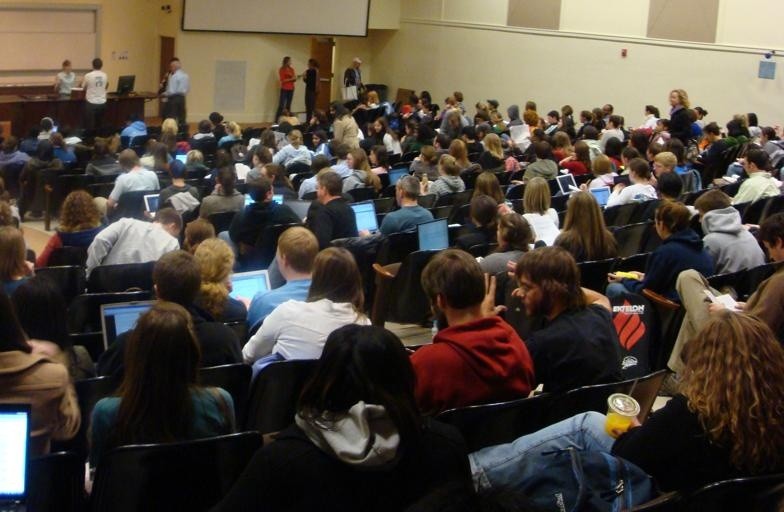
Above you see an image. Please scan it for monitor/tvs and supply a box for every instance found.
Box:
[107,75,136,96]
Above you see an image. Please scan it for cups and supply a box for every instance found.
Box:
[604,393,641,440]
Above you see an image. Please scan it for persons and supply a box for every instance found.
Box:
[521,177,560,249]
[87,208,181,282]
[37,117,53,140]
[120,115,146,136]
[592,108,606,133]
[390,90,440,123]
[543,111,561,136]
[271,129,312,168]
[768,167,784,195]
[454,194,497,248]
[328,174,435,257]
[204,150,238,191]
[368,91,380,109]
[258,164,299,199]
[643,105,660,128]
[307,168,355,218]
[159,57,179,119]
[621,149,641,175]
[207,324,478,512]
[217,178,303,272]
[329,140,341,166]
[303,58,320,123]
[562,105,573,116]
[36,191,103,271]
[628,133,646,154]
[744,198,784,264]
[330,101,359,149]
[246,130,278,163]
[628,171,683,224]
[506,104,522,127]
[694,189,765,276]
[649,119,670,145]
[472,133,505,170]
[17,139,65,217]
[93,250,244,384]
[605,137,622,167]
[522,129,545,162]
[232,144,251,181]
[665,90,690,139]
[278,109,300,126]
[54,60,76,95]
[331,144,354,180]
[475,212,534,277]
[552,191,619,263]
[577,111,596,139]
[420,153,466,199]
[342,149,382,193]
[308,130,331,161]
[236,226,318,329]
[344,57,365,97]
[368,146,392,175]
[185,219,215,251]
[688,109,701,139]
[449,139,482,189]
[663,138,690,174]
[407,249,536,418]
[400,119,418,154]
[275,56,296,122]
[409,145,440,181]
[82,58,108,136]
[308,110,329,131]
[503,151,521,173]
[0,201,19,227]
[526,101,536,111]
[139,139,156,168]
[556,117,575,137]
[687,123,721,173]
[157,119,178,152]
[723,119,750,148]
[409,125,436,154]
[580,155,619,191]
[599,115,625,152]
[524,110,540,136]
[20,125,41,154]
[433,134,448,153]
[759,127,784,167]
[352,90,371,114]
[162,60,188,123]
[551,131,573,161]
[374,118,402,156]
[11,278,97,384]
[184,150,207,172]
[746,113,761,138]
[506,141,558,195]
[0,137,32,170]
[0,225,34,280]
[606,199,716,349]
[190,237,248,323]
[246,146,273,184]
[660,210,784,396]
[314,172,359,250]
[482,246,622,394]
[606,157,658,207]
[582,126,601,156]
[696,107,707,129]
[85,141,123,175]
[76,129,96,171]
[209,112,228,143]
[728,143,759,177]
[242,247,372,359]
[620,117,630,142]
[87,302,235,498]
[93,148,160,228]
[152,144,169,169]
[729,148,779,205]
[468,309,784,495]
[200,166,245,219]
[298,154,331,199]
[242,127,255,145]
[0,285,81,458]
[603,104,613,125]
[654,152,677,178]
[0,178,10,203]
[559,140,592,176]
[469,172,505,207]
[176,119,216,152]
[49,132,74,169]
[218,121,242,148]
[646,144,662,161]
[144,160,202,221]
[440,91,508,133]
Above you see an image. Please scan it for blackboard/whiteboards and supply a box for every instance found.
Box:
[0,5,102,87]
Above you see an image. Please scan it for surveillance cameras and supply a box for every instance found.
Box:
[762,50,775,61]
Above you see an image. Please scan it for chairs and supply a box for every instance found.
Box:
[548,179,560,197]
[88,261,155,292]
[512,199,524,214]
[395,88,415,104]
[578,258,614,297]
[506,184,526,198]
[627,490,684,512]
[388,153,402,163]
[43,173,96,231]
[614,253,649,276]
[688,473,784,511]
[244,358,316,428]
[376,225,417,265]
[574,173,593,186]
[89,430,265,512]
[117,191,156,219]
[602,200,635,226]
[401,151,420,161]
[370,197,398,212]
[434,189,474,206]
[206,211,234,233]
[614,220,651,253]
[435,393,552,451]
[392,162,411,168]
[560,370,667,425]
[33,264,86,294]
[364,84,386,101]
[69,289,152,332]
[450,203,470,226]
[746,261,784,295]
[681,168,703,191]
[28,450,86,512]
[642,268,748,370]
[87,183,111,194]
[255,223,297,260]
[494,272,508,316]
[390,248,442,322]
[639,198,664,222]
[690,201,752,232]
[197,364,252,426]
[470,243,498,256]
[744,195,784,224]
[330,236,375,310]
[56,375,119,463]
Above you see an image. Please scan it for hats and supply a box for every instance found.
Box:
[487,99,498,106]
[508,105,519,120]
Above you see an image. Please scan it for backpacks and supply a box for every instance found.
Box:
[609,290,653,381]
[472,446,657,512]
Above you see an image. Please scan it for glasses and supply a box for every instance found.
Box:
[401,173,410,189]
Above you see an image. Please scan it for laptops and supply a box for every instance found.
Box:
[416,217,449,252]
[344,200,379,237]
[229,270,271,312]
[387,167,409,185]
[588,186,610,207]
[53,126,58,132]
[176,155,187,164]
[0,403,32,499]
[144,194,161,217]
[100,300,160,352]
[556,173,579,195]
[244,194,284,205]
